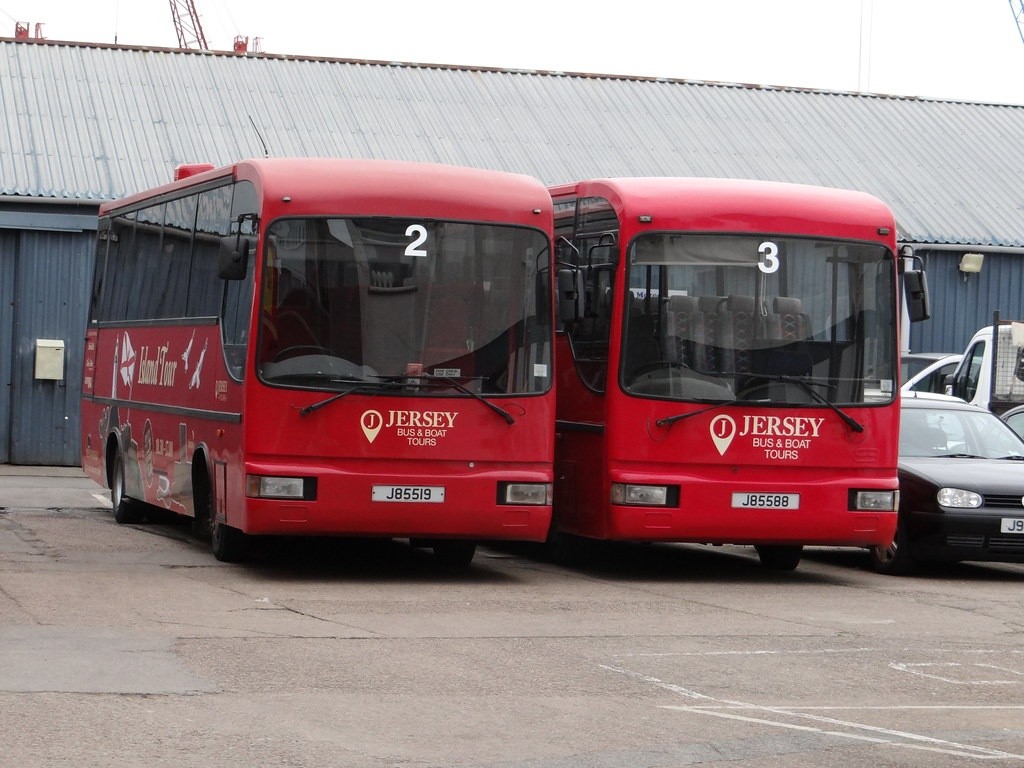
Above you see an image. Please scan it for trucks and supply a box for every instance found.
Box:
[943,311,1024,414]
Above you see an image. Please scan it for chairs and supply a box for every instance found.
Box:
[568,292,814,392]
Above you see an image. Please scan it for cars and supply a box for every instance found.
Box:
[870,390,1024,570]
[863,352,1023,402]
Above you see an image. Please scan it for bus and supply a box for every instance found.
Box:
[547,176,932,574]
[80,157,583,568]
